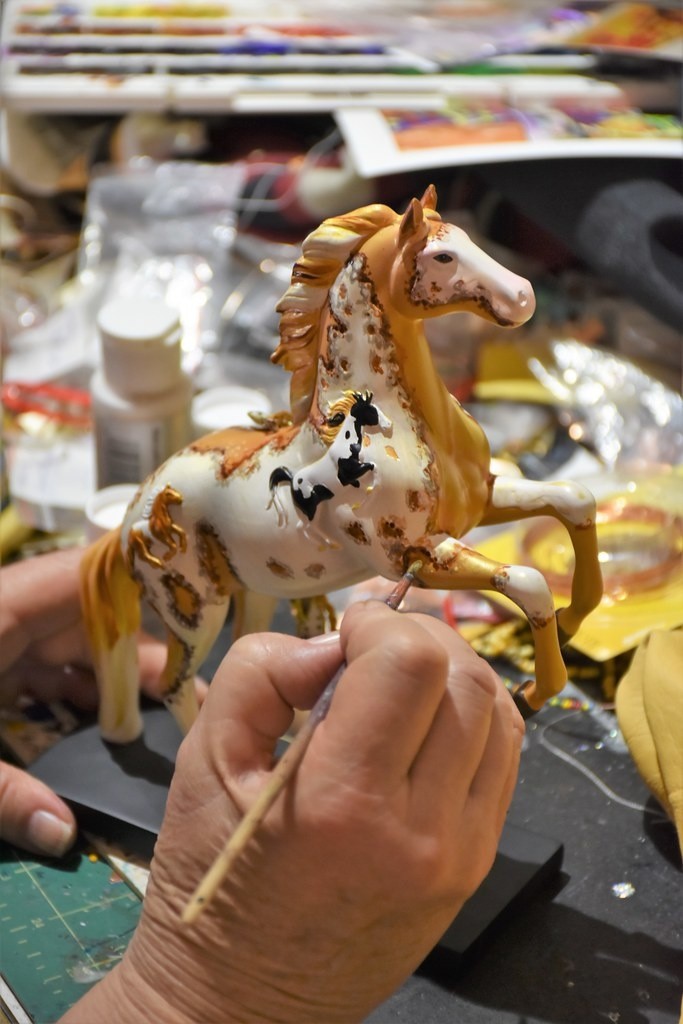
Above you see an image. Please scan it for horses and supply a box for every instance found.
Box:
[264,388,395,553]
[77,182,602,773]
[124,484,188,579]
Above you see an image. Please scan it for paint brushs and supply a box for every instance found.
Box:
[175,558,425,931]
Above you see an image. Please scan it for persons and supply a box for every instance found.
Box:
[0,531,524,1024]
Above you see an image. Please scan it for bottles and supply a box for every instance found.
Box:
[90,298,192,483]
